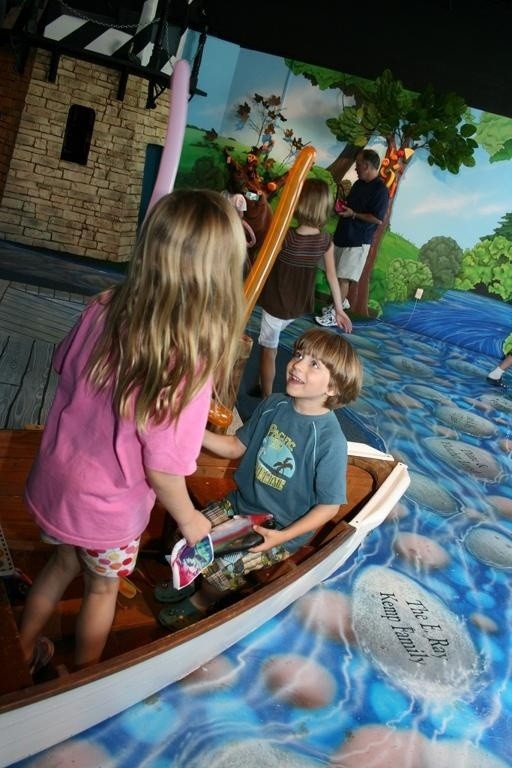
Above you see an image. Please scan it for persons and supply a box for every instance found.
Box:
[314,149,390,327]
[16,188,257,677]
[257,177,353,401]
[219,172,249,221]
[153,327,364,633]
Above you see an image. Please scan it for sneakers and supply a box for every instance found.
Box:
[486,367,504,385]
[314,298,350,327]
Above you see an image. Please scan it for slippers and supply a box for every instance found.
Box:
[155,580,205,631]
[24,637,54,676]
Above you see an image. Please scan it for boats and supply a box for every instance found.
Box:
[0,425,411,768]
